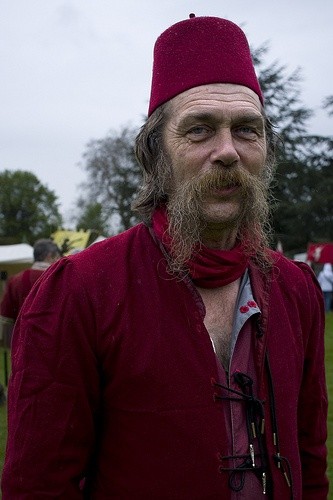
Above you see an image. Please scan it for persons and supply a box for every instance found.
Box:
[0,239,59,353]
[317,263,333,312]
[0,15,332,499]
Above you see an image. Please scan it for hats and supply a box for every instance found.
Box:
[146,12,262,105]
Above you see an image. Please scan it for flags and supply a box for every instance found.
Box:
[306,241,332,264]
